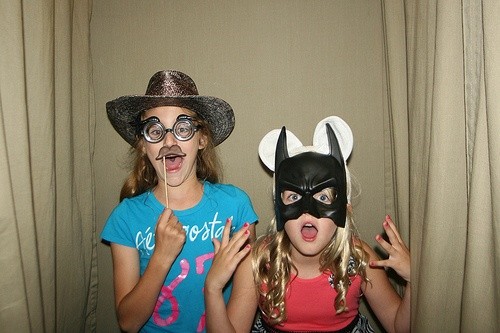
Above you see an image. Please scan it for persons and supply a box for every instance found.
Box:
[204,145,410,333]
[102,70,258,332]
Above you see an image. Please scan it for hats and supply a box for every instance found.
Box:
[106,71,235,150]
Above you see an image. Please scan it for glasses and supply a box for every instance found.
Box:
[135,116,206,143]
